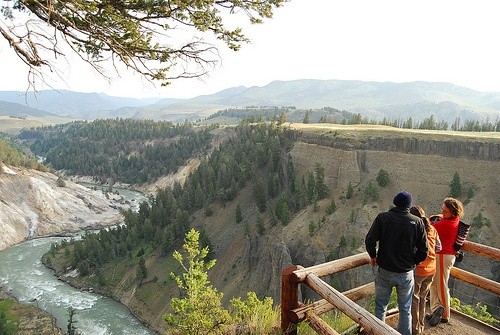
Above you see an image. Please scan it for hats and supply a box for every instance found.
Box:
[393,192,412,208]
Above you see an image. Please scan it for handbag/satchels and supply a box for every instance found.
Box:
[454,221,470,250]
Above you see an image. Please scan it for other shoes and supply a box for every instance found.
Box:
[428,307,443,326]
[426,314,447,323]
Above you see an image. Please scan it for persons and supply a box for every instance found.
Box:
[426,198,464,326]
[410,205,442,335]
[365,191,428,335]
